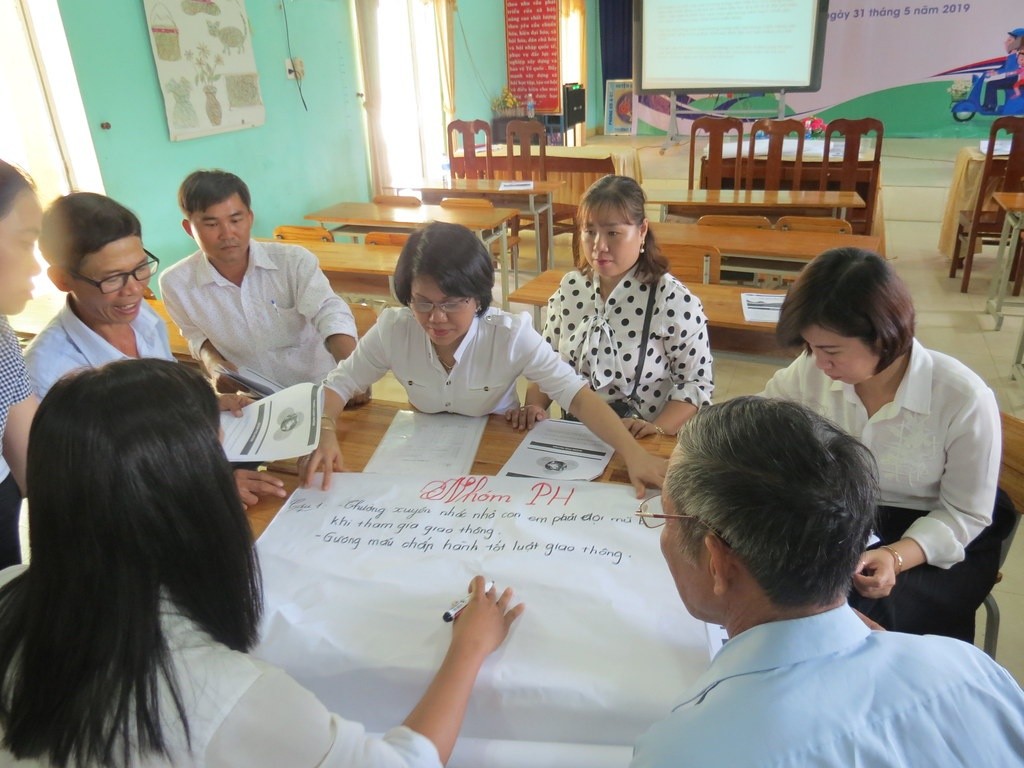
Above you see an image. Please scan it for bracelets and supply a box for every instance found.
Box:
[881,546,903,575]
[655,426,664,434]
[321,416,336,431]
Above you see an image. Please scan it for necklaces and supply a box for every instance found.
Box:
[437,354,454,369]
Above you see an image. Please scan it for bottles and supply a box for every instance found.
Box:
[804,120,812,139]
[526,94,534,119]
[751,120,764,141]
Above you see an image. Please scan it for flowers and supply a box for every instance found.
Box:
[490,85,519,116]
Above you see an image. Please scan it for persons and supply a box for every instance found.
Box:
[506,175,715,440]
[629,395,1024,768]
[297,220,669,500]
[0,160,287,572]
[158,168,371,406]
[0,358,526,768]
[753,246,1016,646]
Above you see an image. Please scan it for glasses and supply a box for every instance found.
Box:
[406,294,472,313]
[634,493,735,551]
[62,246,160,294]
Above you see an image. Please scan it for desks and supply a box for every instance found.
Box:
[238,182,889,364]
[456,143,639,217]
[984,189,1024,331]
[697,140,882,225]
[181,400,713,721]
[936,142,1023,266]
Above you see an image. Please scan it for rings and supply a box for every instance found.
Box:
[520,406,525,411]
[302,457,311,461]
[640,420,645,423]
[859,560,865,566]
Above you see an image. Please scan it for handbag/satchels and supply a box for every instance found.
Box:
[562,402,648,424]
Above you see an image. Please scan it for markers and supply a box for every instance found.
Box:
[443,580,495,622]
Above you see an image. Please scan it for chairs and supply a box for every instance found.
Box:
[270,106,885,290]
[946,116,1024,292]
[974,409,1024,658]
